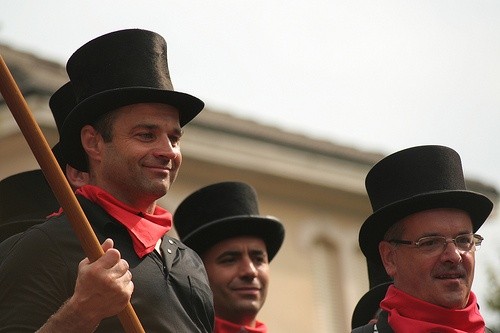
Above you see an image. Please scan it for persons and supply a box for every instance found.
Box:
[0,29,216,333]
[172,181,286,333]
[351,145,495,333]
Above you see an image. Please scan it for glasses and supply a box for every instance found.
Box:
[379,233,485,257]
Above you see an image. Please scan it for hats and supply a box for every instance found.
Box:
[48,29,205,172]
[173,180,285,263]
[357,145,496,263]
